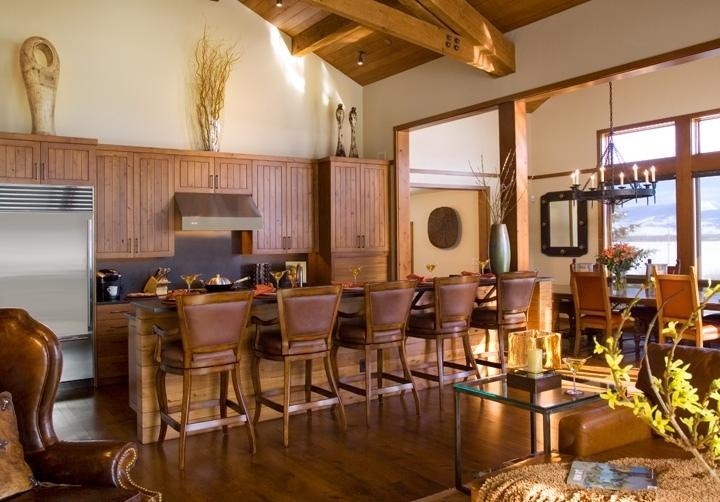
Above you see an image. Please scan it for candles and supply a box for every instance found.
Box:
[632,164,639,181]
[590,174,596,188]
[643,169,650,183]
[618,171,625,185]
[575,169,581,184]
[571,173,575,185]
[598,165,605,182]
[650,165,656,182]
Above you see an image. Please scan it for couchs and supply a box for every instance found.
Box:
[0,305,162,502]
[472,342,720,501]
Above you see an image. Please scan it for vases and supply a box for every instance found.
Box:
[594,243,648,291]
[610,271,627,296]
[490,223,511,274]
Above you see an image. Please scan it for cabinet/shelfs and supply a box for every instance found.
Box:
[94,143,176,260]
[177,151,252,196]
[312,253,398,288]
[249,155,321,254]
[95,302,134,391]
[0,132,97,186]
[316,155,396,253]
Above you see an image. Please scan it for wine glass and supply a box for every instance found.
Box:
[270,270,288,289]
[562,359,588,397]
[180,274,200,294]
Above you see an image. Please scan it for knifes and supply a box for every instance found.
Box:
[154,267,172,280]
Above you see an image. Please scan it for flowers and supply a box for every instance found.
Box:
[467,147,537,223]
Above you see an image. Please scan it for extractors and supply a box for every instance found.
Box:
[173,193,264,231]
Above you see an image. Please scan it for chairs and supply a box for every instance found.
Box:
[459,271,537,383]
[646,259,681,291]
[401,276,484,423]
[152,290,258,472]
[568,263,640,359]
[330,286,421,428]
[252,284,348,448]
[649,265,719,348]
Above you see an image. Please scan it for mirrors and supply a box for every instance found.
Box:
[540,190,589,257]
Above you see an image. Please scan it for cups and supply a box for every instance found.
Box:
[107,286,118,296]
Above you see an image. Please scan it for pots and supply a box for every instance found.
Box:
[205,274,250,292]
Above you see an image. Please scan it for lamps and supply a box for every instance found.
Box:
[274,0,283,8]
[358,52,363,66]
[570,81,658,213]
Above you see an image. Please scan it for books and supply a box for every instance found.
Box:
[566,460,658,493]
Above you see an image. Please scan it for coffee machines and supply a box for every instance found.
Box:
[97,269,122,303]
